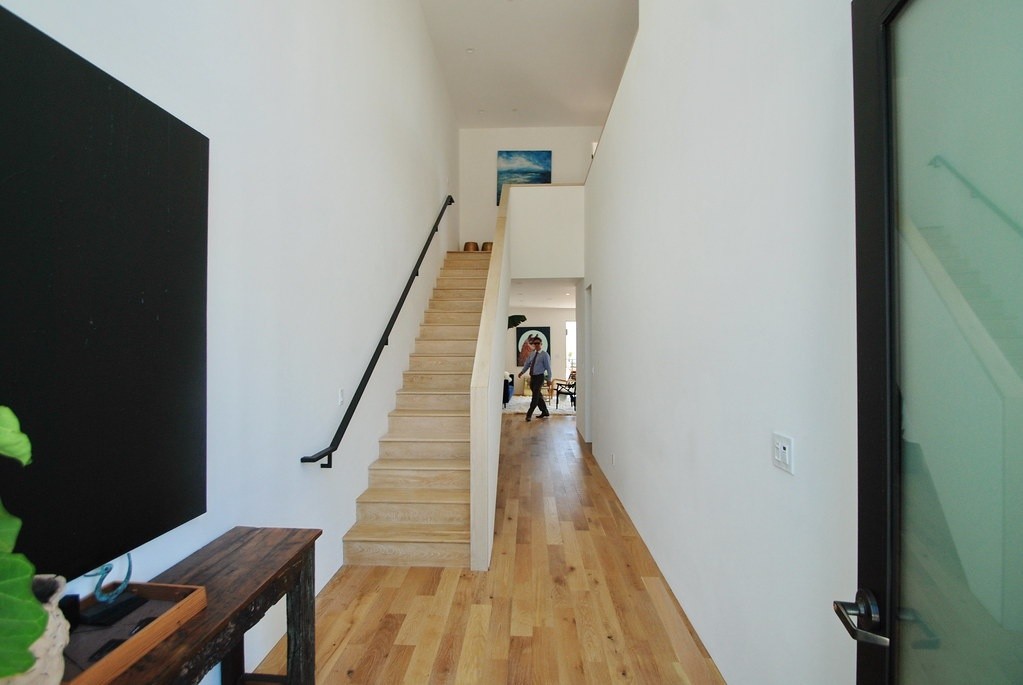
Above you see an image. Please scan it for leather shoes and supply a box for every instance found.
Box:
[536,410,549,418]
[526,415,531,421]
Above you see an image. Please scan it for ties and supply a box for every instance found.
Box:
[530,352,538,375]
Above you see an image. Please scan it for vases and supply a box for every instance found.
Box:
[464,242,479,251]
[481,241,493,251]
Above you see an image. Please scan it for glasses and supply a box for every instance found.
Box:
[534,342,541,345]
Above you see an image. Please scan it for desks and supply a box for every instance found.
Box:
[111,526,323,685]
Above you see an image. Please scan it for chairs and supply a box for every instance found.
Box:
[556,381,577,411]
[551,370,576,399]
[541,383,551,406]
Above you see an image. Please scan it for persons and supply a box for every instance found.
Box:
[517,337,553,422]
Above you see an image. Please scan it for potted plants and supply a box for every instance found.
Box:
[0,403,73,685]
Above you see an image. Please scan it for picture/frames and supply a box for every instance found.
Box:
[496,150,553,206]
[516,326,551,366]
